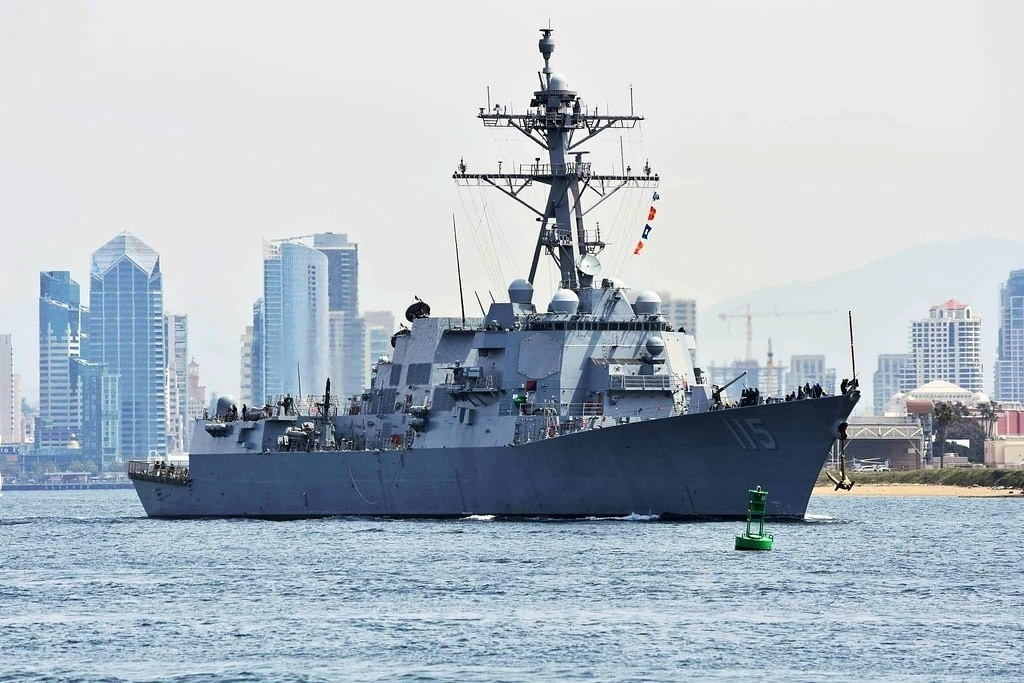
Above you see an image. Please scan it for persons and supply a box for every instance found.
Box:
[786,382,822,402]
[741,387,771,407]
[714,400,739,411]
[841,379,848,394]
[231,403,247,421]
[149,461,175,477]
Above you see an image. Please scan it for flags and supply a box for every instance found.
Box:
[634,206,657,254]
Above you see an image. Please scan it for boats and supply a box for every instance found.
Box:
[129,22,861,520]
